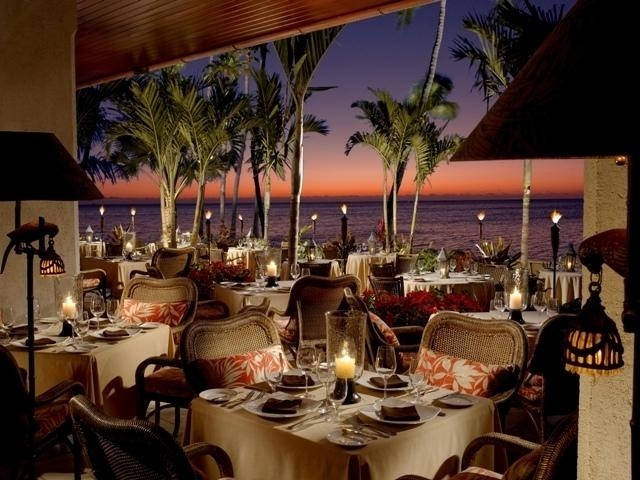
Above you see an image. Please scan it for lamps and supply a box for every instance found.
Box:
[0,131,104,417]
[564,227,626,378]
[450,0,640,480]
[565,242,576,272]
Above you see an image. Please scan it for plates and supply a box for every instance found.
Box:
[198,361,480,448]
[4,316,159,354]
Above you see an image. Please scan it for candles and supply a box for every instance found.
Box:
[62,292,78,320]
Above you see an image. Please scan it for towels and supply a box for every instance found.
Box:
[262,398,302,414]
[382,405,420,421]
[9,325,37,336]
[25,338,56,346]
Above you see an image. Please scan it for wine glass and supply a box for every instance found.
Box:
[254,263,311,288]
[489,291,561,320]
[0,295,120,346]
[356,242,384,255]
[408,258,478,279]
[263,339,429,424]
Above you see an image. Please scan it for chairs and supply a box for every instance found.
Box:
[497,314,578,445]
[0,344,84,480]
[66,394,235,480]
[392,428,570,480]
